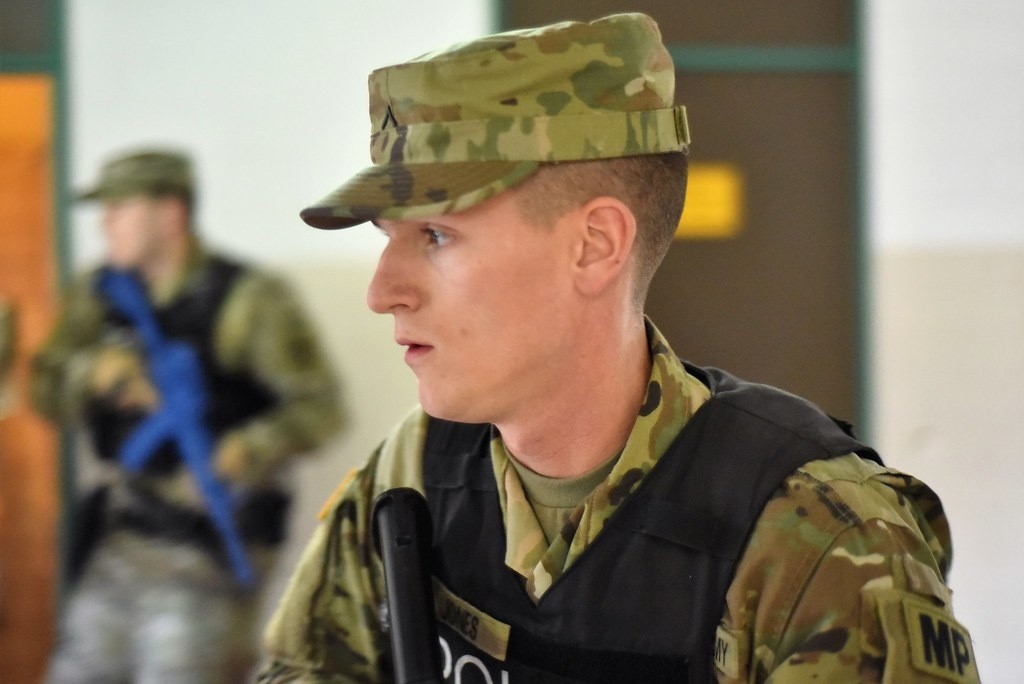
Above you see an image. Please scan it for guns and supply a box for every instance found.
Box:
[90,260,257,585]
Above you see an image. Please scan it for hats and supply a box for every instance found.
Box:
[80,151,190,199]
[300,12,692,231]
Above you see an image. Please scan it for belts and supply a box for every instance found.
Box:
[121,497,255,540]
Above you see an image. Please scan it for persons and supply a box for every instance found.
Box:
[26,151,346,684]
[244,13,981,684]
